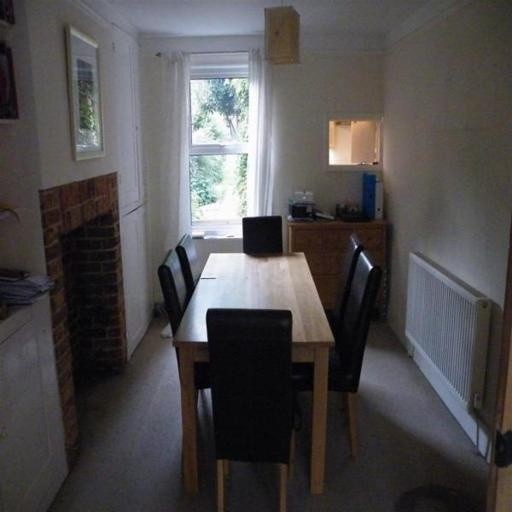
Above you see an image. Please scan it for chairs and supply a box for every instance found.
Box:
[158,249,212,478]
[295,251,382,465]
[324,233,364,328]
[206,309,295,512]
[242,216,282,253]
[175,234,204,292]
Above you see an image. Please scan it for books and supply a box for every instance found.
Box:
[0,267,56,309]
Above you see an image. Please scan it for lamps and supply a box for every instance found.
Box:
[264,7,300,65]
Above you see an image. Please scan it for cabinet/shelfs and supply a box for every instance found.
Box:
[286,216,388,323]
[0,314,71,512]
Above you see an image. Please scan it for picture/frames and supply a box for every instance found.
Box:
[63,24,105,163]
[0,0,16,28]
[0,42,20,122]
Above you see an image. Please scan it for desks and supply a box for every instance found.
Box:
[172,252,335,496]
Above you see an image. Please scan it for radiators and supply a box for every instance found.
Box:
[404,249,493,414]
